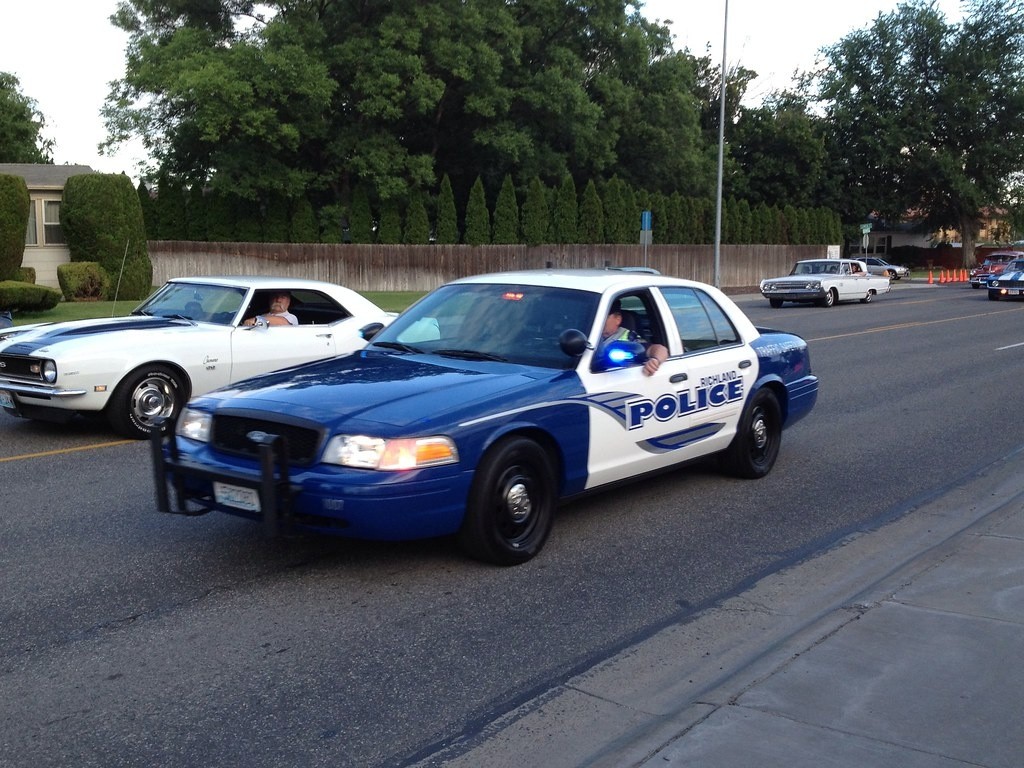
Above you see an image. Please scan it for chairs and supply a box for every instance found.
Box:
[619,310,643,338]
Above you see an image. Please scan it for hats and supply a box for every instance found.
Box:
[590,294,621,314]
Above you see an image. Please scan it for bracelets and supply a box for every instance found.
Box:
[649,356,662,365]
[253,317,257,326]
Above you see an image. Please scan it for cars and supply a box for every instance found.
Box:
[0,274,441,439]
[149,270,820,567]
[987,259,1024,300]
[760,259,891,307]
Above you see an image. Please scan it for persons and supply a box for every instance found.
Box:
[222,290,299,328]
[830,264,840,274]
[851,264,866,276]
[591,298,668,375]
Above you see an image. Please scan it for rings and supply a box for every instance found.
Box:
[247,324,250,325]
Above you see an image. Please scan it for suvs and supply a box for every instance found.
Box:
[968,251,1024,289]
[854,256,910,279]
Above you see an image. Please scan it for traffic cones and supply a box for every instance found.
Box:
[928,270,934,284]
[938,269,969,283]
